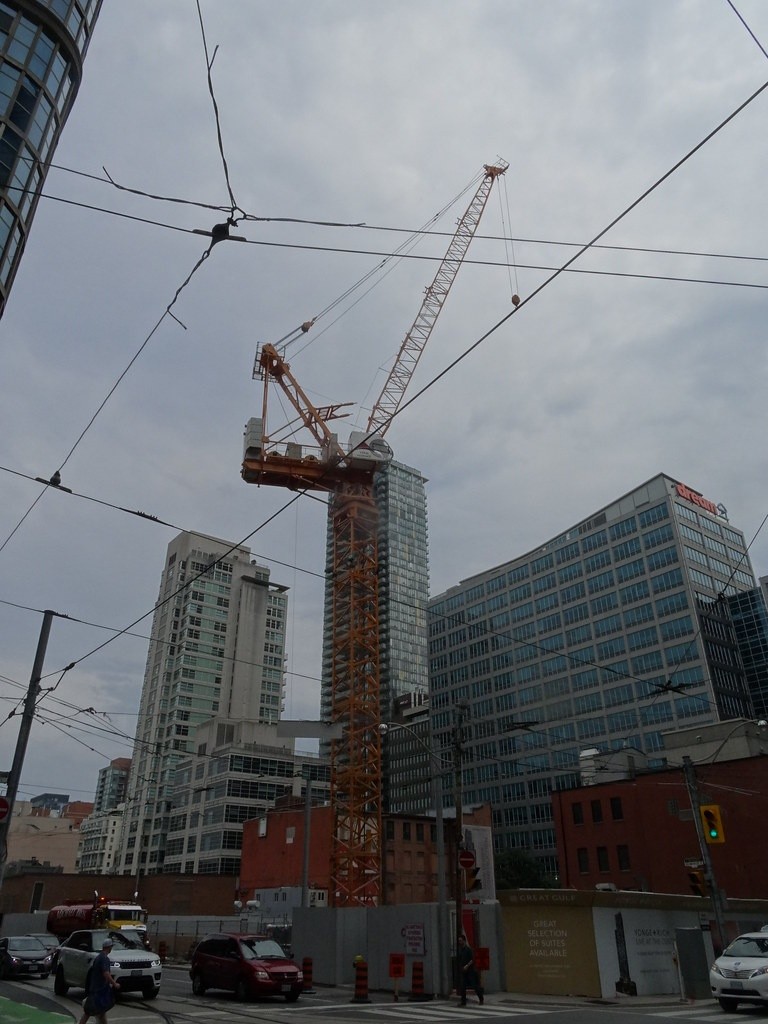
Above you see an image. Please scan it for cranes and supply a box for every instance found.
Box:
[238,156,528,461]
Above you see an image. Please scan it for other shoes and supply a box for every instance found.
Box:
[461,999,466,1005]
[479,997,485,1005]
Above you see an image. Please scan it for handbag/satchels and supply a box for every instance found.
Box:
[84,986,115,1016]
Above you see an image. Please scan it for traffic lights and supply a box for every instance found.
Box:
[699,802,725,845]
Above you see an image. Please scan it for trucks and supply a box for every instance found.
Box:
[46,890,151,951]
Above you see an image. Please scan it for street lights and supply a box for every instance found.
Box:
[678,719,767,955]
[377,719,465,939]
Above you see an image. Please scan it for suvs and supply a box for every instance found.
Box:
[53,926,162,1002]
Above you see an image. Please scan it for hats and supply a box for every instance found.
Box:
[102,938,114,948]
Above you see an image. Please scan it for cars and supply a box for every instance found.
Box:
[190,932,305,1004]
[0,936,52,979]
[23,933,61,958]
[708,925,768,1011]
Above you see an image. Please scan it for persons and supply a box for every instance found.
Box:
[456,934,484,1006]
[78,939,120,1024]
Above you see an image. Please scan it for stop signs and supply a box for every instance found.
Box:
[457,853,475,867]
